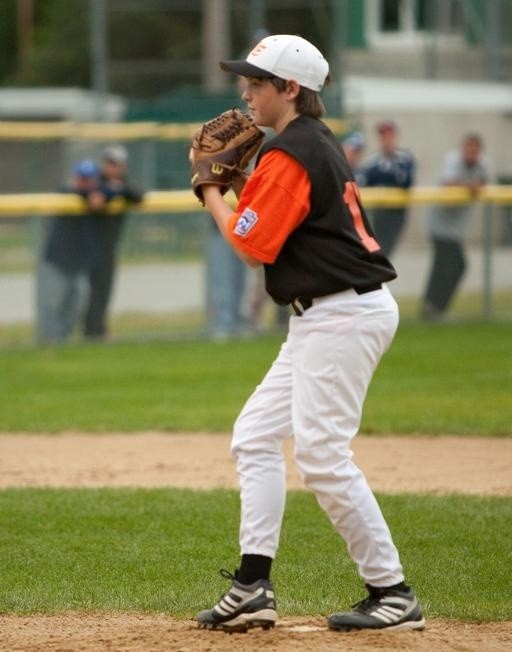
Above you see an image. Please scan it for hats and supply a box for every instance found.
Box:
[221,34,329,96]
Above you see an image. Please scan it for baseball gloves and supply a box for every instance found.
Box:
[184,104,266,206]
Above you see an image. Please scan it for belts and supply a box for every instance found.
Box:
[282,281,384,319]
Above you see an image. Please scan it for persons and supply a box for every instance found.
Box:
[341,133,373,184]
[80,144,145,338]
[205,208,259,343]
[188,34,428,636]
[413,134,488,320]
[358,120,417,262]
[32,158,102,342]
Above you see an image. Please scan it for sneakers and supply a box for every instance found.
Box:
[195,577,278,632]
[327,587,426,632]
